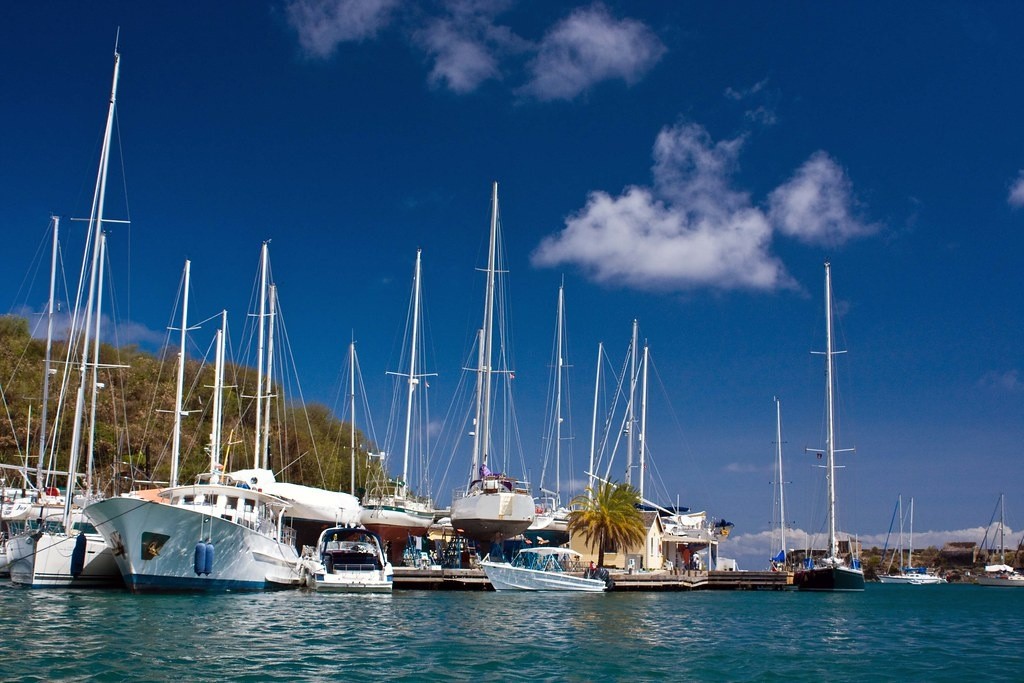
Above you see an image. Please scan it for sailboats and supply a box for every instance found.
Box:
[0,21,869,596]
[976,493,1024,586]
[876,492,950,585]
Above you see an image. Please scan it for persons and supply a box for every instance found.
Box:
[589,560,598,577]
[479,463,486,479]
[561,553,572,572]
[692,551,700,571]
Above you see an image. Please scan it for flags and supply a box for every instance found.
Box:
[768,550,786,564]
[424,381,429,388]
[509,371,515,380]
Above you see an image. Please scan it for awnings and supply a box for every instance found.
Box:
[661,534,719,572]
